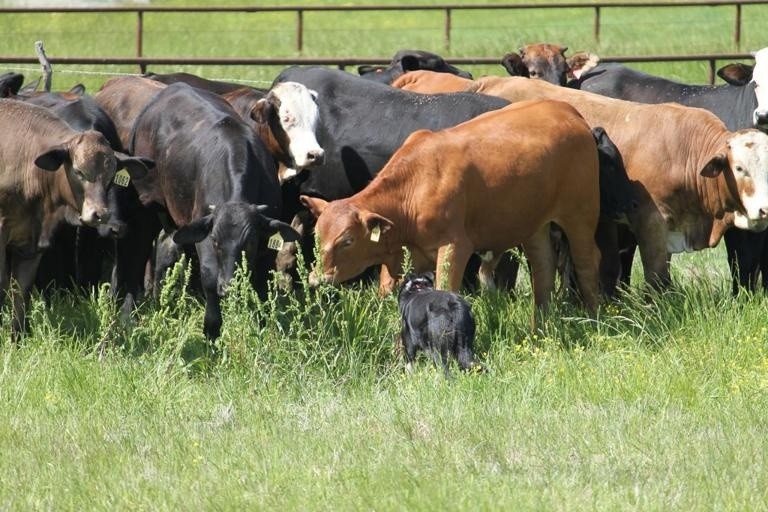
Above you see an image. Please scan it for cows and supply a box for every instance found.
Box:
[298,96,611,340]
[0,42,768,356]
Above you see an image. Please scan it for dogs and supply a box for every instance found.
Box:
[397,271,490,386]
